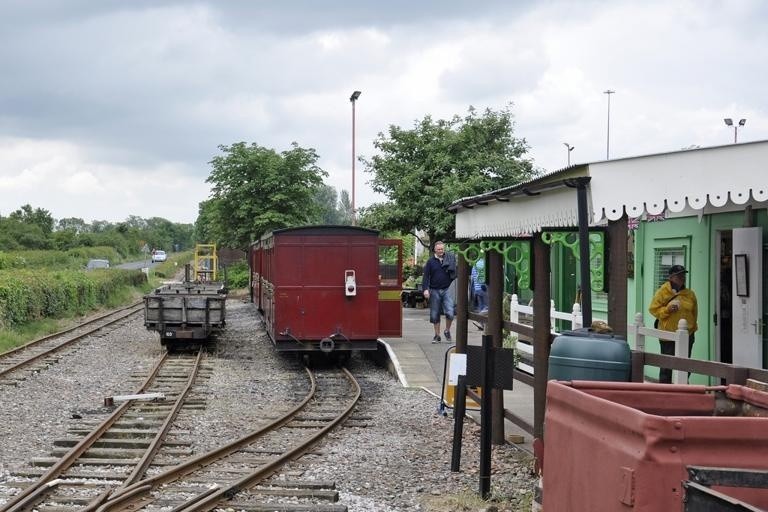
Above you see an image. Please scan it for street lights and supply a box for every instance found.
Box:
[349,91,361,226]
[723,119,747,144]
[603,90,615,160]
[563,143,574,166]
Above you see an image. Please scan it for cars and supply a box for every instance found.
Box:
[152,250,167,263]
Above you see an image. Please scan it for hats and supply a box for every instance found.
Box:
[669,265,688,276]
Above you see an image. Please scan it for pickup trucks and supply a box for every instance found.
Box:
[86,259,113,269]
[403,283,428,307]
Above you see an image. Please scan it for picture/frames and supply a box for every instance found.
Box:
[733,252,750,297]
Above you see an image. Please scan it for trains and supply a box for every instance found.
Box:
[143,243,228,348]
[245,223,403,360]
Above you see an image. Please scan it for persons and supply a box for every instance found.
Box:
[471,258,486,309]
[648,265,699,384]
[422,240,458,345]
[472,279,488,330]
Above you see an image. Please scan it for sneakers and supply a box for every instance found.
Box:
[444,330,451,341]
[432,335,441,343]
[472,321,483,330]
